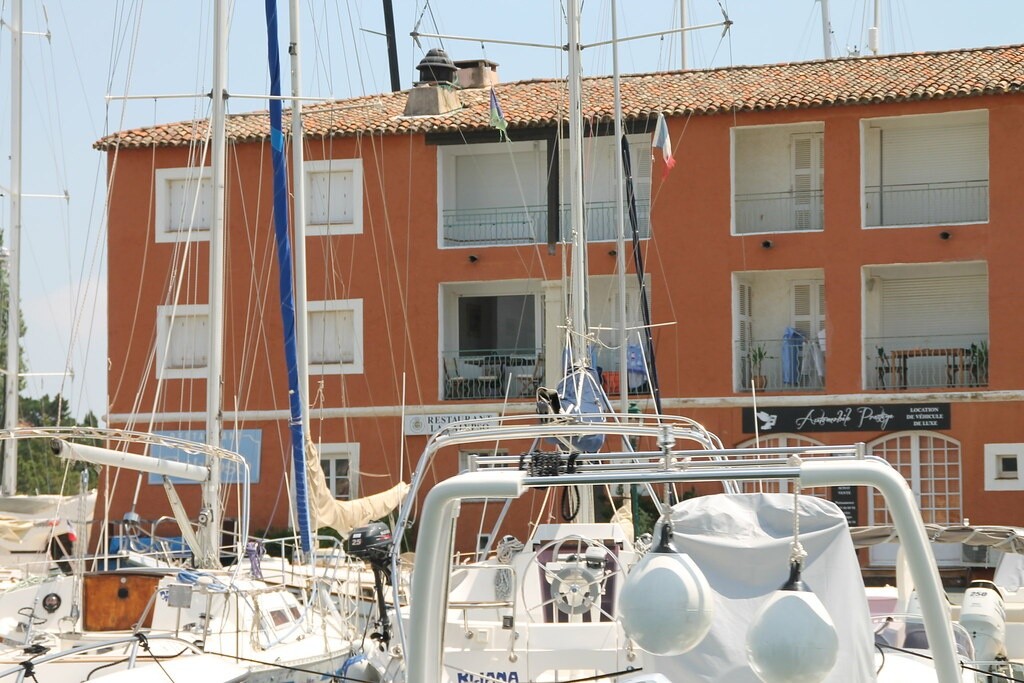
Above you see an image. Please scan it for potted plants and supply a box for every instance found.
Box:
[751,343,768,391]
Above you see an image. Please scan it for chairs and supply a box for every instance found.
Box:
[946,343,978,387]
[874,346,908,391]
[444,353,544,399]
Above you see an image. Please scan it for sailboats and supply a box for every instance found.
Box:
[1,0,1024,683]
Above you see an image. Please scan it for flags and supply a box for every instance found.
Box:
[652,112,677,168]
[488,87,508,131]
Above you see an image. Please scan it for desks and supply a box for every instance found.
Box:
[466,356,537,397]
[891,348,974,391]
